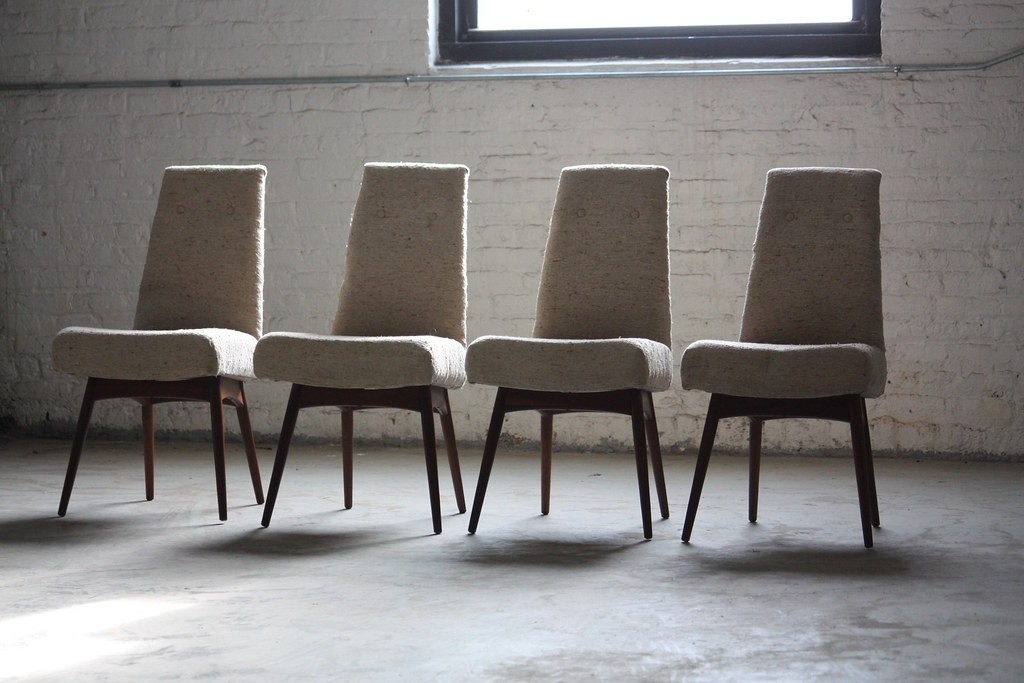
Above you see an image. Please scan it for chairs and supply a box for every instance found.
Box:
[463,164,674,541]
[253,161,473,534]
[680,168,888,549]
[49,164,268,525]
